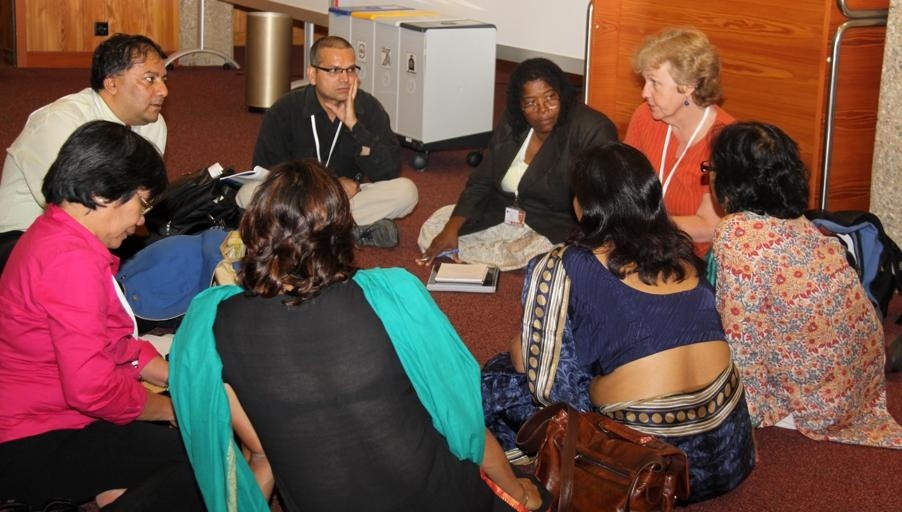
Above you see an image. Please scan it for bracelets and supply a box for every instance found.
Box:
[249,450,267,460]
[517,478,531,508]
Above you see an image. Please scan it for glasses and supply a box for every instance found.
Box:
[131,187,156,216]
[519,92,561,114]
[699,159,716,173]
[309,63,362,77]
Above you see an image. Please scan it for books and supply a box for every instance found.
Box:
[219,165,272,184]
[428,260,499,295]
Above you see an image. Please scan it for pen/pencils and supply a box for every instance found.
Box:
[420,249,460,262]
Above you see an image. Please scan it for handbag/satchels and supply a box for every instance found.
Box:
[532,406,693,512]
[144,162,244,239]
[808,208,894,327]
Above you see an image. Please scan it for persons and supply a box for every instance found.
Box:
[235,35,419,249]
[415,55,618,269]
[704,122,902,451]
[482,139,756,507]
[623,25,739,258]
[213,161,556,511]
[0,120,209,511]
[0,34,170,275]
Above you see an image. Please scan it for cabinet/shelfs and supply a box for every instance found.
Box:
[12,0,180,69]
[581,0,890,220]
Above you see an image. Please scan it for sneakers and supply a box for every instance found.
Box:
[350,217,401,251]
